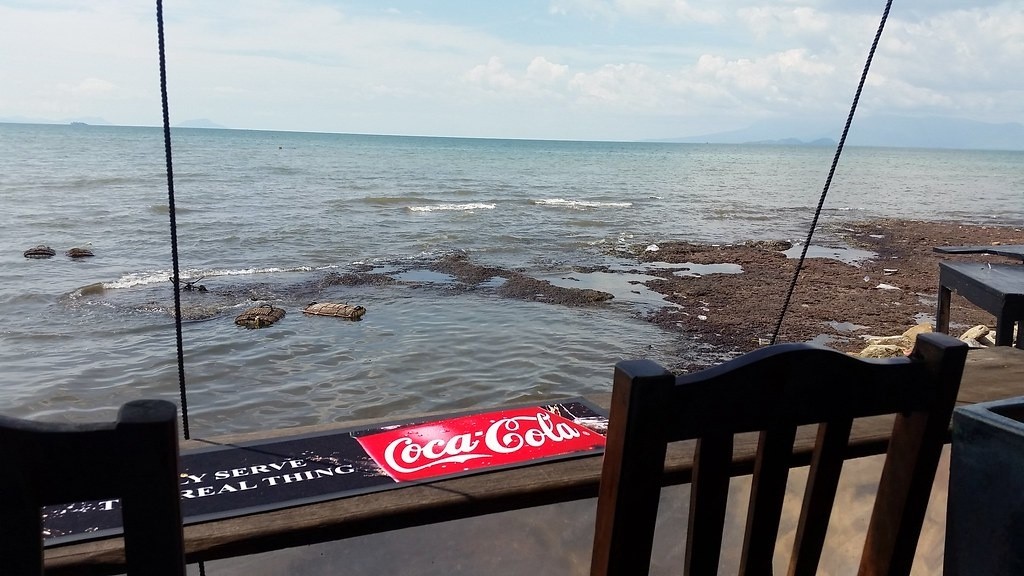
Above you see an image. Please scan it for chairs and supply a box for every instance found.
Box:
[0,400,186,576]
[590,331,970,576]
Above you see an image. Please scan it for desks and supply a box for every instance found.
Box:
[931,244,1024,349]
[42,345,1024,576]
